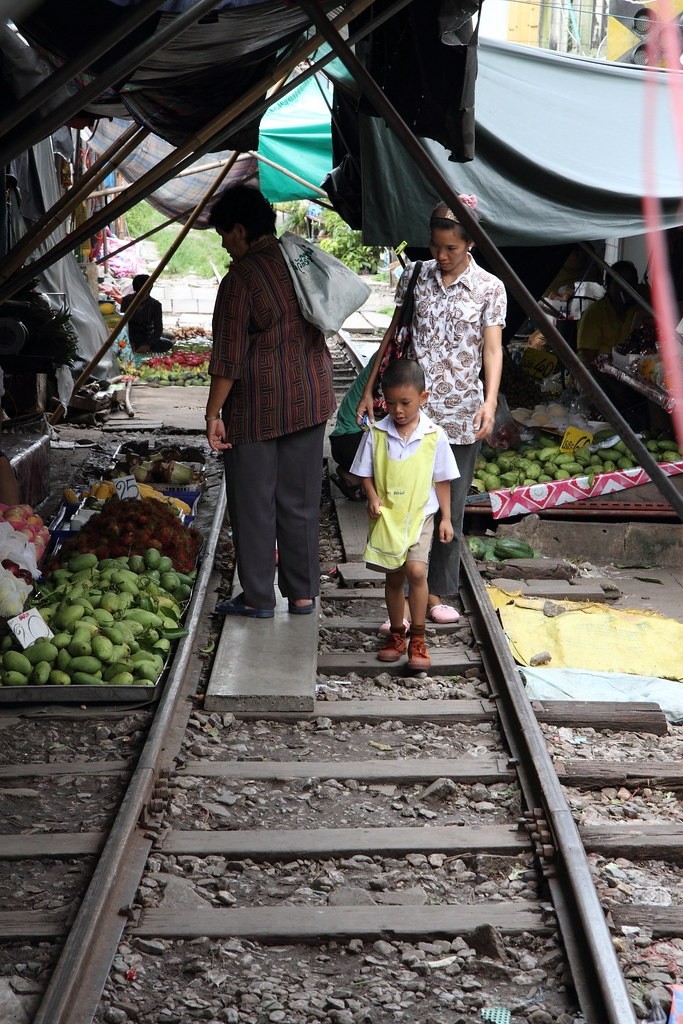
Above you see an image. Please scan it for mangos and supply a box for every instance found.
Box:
[0,549,197,687]
[456,427,682,493]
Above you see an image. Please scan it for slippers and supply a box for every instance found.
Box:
[427,604,459,624]
[287,597,316,614]
[329,472,366,501]
[216,592,276,618]
[379,618,409,635]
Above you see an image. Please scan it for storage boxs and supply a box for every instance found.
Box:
[611,345,657,376]
[33,444,207,585]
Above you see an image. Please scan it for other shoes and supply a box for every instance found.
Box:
[408,638,432,670]
[377,635,407,662]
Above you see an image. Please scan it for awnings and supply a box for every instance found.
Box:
[0,0,683,434]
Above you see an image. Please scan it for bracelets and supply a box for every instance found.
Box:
[205,414,220,422]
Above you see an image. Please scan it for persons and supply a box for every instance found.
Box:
[348,358,461,670]
[205,182,333,619]
[356,195,507,639]
[577,261,651,363]
[329,349,380,501]
[120,275,175,354]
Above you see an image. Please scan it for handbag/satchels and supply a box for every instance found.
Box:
[276,228,372,340]
[370,258,424,413]
